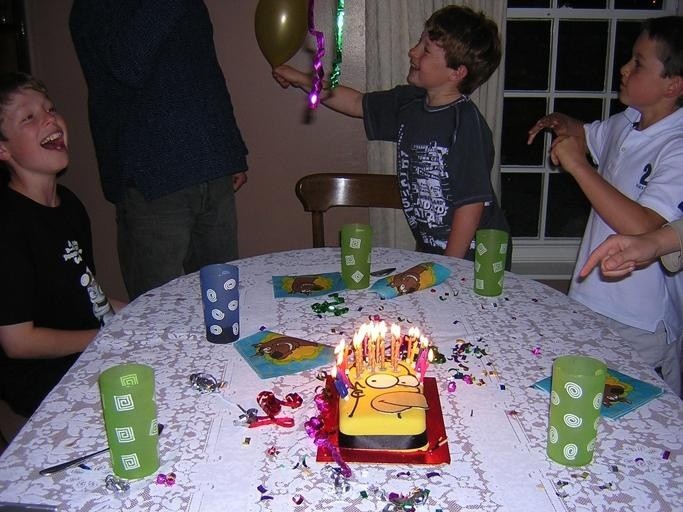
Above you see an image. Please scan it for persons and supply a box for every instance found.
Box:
[69,0,249,301]
[578,218,683,281]
[272,4,513,272]
[0,72,131,418]
[526,15,683,396]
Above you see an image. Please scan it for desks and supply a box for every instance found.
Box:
[0,247,683,512]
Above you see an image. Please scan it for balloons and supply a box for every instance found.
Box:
[253,0,309,69]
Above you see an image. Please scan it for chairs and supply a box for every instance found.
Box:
[296,173,404,248]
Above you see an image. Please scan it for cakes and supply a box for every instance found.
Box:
[338,360,431,452]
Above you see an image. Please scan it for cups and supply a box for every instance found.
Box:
[340,222,374,291]
[473,227,510,297]
[544,355,608,468]
[97,363,161,480]
[199,263,240,344]
